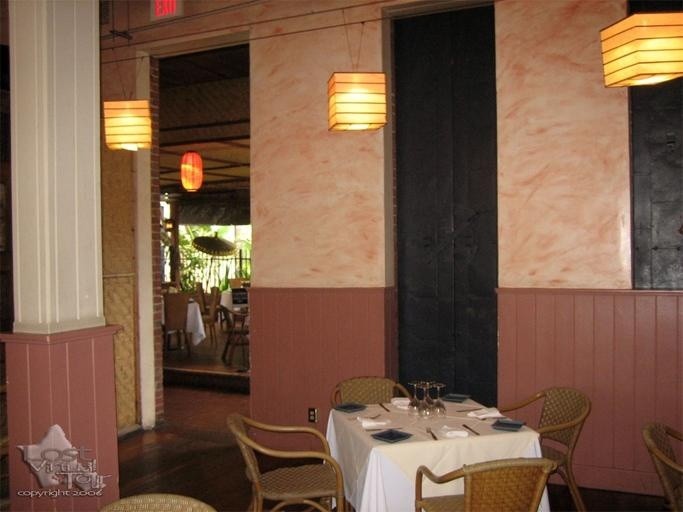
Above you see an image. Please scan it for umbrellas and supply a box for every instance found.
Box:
[192,231,238,293]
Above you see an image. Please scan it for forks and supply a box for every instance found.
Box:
[348,413,380,421]
[425,426,438,440]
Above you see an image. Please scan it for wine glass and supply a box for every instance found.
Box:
[407,380,447,418]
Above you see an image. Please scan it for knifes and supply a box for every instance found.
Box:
[456,408,482,413]
[378,402,389,413]
[462,424,481,435]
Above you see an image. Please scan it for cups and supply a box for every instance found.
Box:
[240,306,248,314]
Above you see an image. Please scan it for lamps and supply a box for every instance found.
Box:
[325,7,389,132]
[103,46,151,151]
[598,0,682,90]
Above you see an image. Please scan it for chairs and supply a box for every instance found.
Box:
[643,418,683,509]
[161,275,251,376]
[329,375,412,412]
[414,457,561,512]
[224,410,345,512]
[98,492,218,512]
[501,384,592,512]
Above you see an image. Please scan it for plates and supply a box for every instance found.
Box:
[333,402,367,413]
[440,393,471,403]
[370,428,411,443]
[491,417,525,431]
[389,397,407,406]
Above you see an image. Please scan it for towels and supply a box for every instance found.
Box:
[356,415,388,426]
[468,407,502,420]
[439,425,467,438]
[390,397,410,410]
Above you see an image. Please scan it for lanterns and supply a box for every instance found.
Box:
[181,151,204,193]
[100,97,162,152]
[324,68,388,134]
[599,10,682,87]
